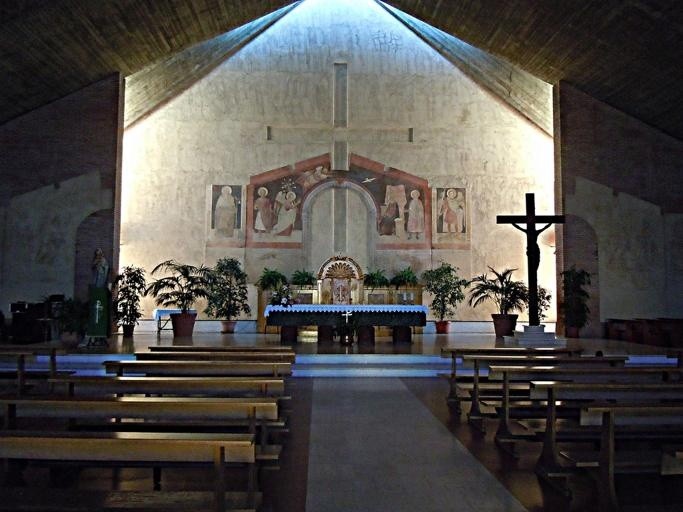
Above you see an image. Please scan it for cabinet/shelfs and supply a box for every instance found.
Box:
[363,283,424,338]
[256,284,318,335]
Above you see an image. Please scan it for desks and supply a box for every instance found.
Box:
[263,304,428,346]
[151,307,195,336]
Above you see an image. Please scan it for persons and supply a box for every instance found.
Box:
[91,247,109,286]
[440,190,463,233]
[215,186,236,236]
[272,191,301,236]
[301,165,332,191]
[407,190,424,240]
[377,193,399,235]
[254,186,273,233]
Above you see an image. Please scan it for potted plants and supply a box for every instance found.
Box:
[140,254,224,339]
[417,260,473,334]
[554,266,592,339]
[109,261,148,338]
[198,255,252,334]
[467,264,530,337]
[46,296,103,347]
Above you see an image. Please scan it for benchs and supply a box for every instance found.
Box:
[435,343,682,512]
[0,336,298,512]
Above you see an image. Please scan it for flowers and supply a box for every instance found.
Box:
[262,278,306,308]
